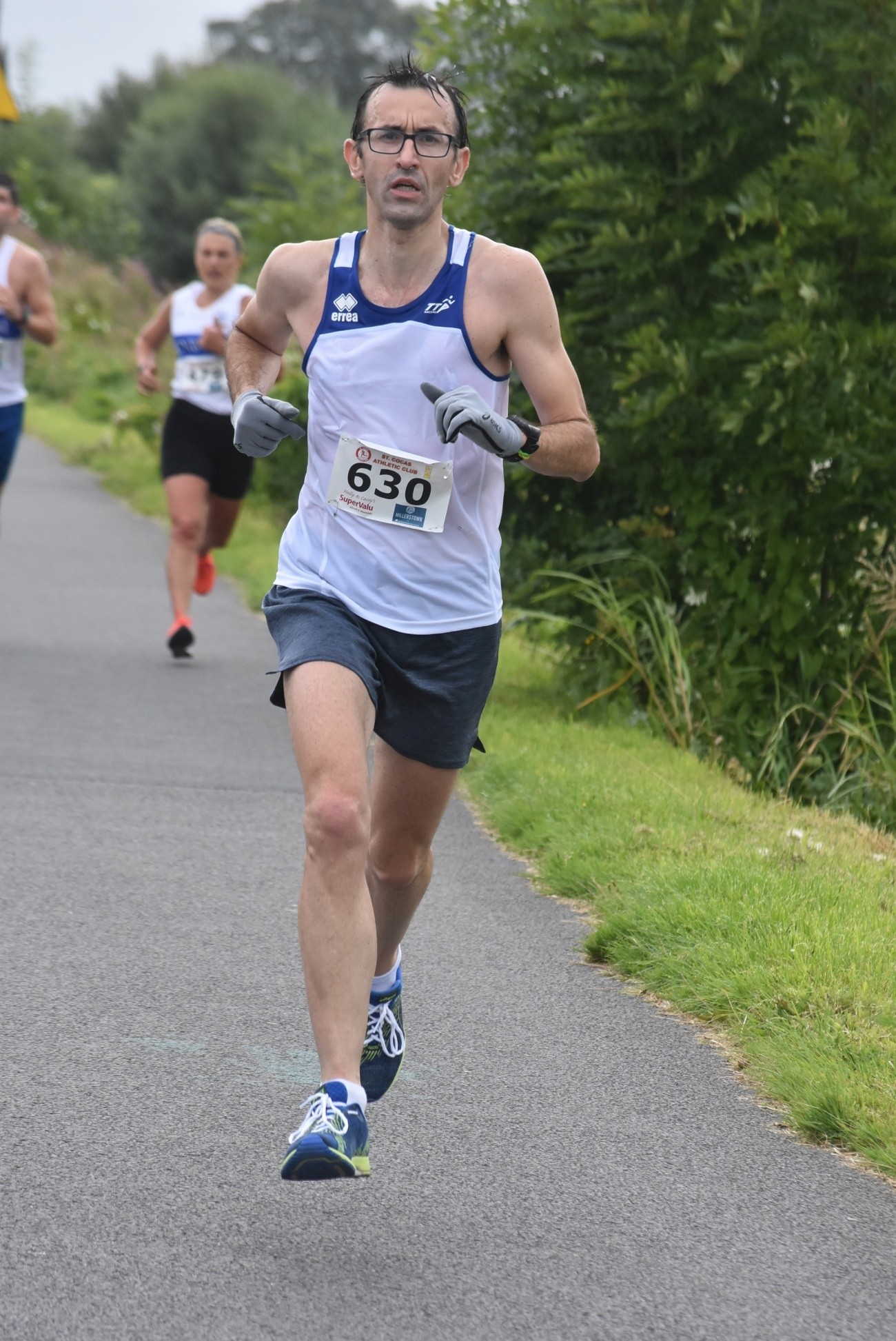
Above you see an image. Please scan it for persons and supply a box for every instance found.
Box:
[132,219,285,658]
[0,173,60,497]
[224,65,603,1179]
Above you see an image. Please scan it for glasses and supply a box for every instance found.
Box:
[197,224,242,252]
[356,127,461,158]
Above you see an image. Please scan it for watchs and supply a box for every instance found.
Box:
[496,414,542,463]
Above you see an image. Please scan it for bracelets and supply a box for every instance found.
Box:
[12,307,28,328]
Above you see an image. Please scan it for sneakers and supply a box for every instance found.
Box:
[359,963,406,1102]
[280,1081,371,1181]
[194,554,215,595]
[167,616,196,658]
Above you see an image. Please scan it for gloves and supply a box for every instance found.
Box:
[420,382,524,457]
[231,390,302,457]
